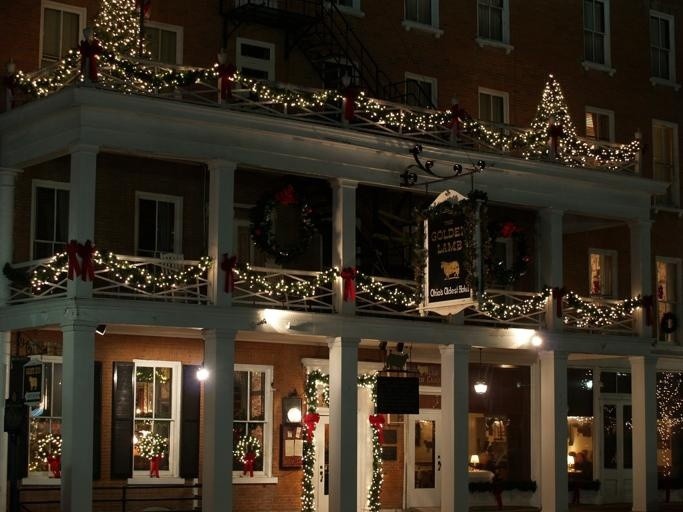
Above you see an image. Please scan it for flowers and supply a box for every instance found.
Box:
[481,220,534,288]
[247,180,318,266]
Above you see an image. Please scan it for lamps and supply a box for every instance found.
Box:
[95,326,106,336]
[469,454,480,469]
[473,348,488,395]
[195,341,209,382]
[567,455,576,472]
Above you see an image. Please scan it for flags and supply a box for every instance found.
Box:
[374,374,420,415]
[426,202,474,305]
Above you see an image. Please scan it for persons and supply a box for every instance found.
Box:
[571,453,592,471]
[472,449,502,474]
[486,446,500,465]
